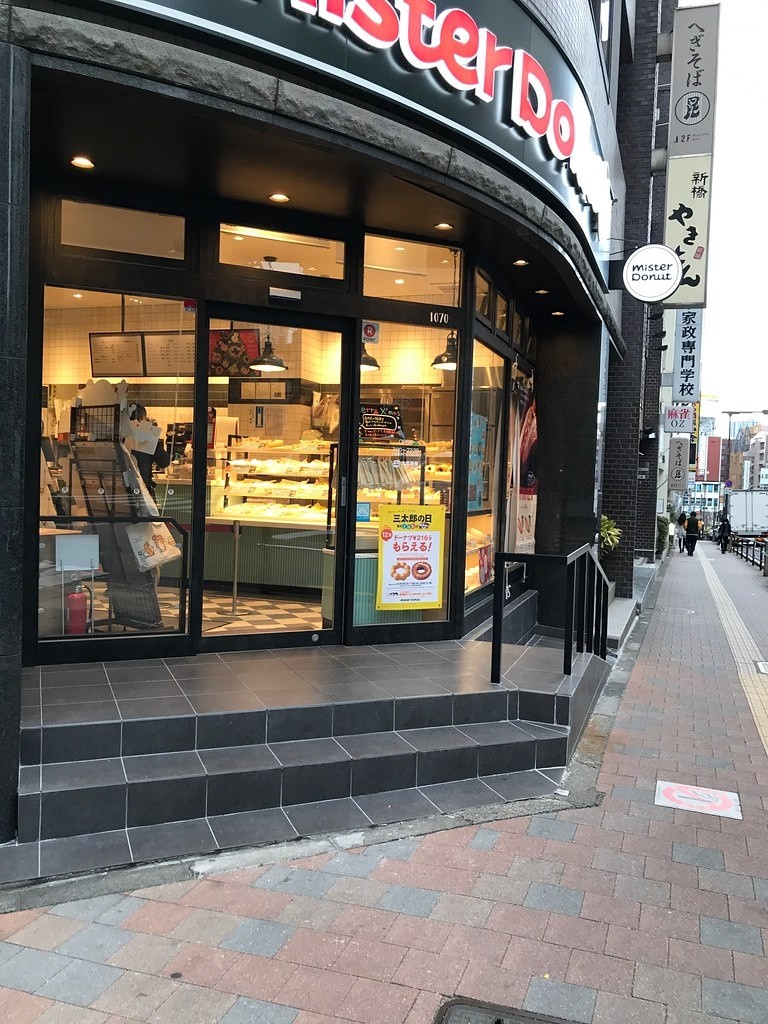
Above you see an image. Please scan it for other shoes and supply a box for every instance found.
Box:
[688,553,693,556]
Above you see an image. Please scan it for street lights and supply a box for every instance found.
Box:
[720,409,768,522]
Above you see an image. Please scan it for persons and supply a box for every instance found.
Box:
[105,403,170,596]
[675,513,686,553]
[684,511,702,556]
[718,519,731,554]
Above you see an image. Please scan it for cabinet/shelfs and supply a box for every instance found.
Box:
[224,435,453,523]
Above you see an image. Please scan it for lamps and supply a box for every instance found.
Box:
[431,331,456,370]
[361,342,380,371]
[249,325,288,372]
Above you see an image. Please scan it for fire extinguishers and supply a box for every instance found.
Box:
[64,580,92,634]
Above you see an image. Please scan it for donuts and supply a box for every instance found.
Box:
[424,464,453,473]
[411,562,432,580]
[390,562,411,581]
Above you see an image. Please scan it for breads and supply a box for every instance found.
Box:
[358,485,441,498]
[424,440,452,452]
[466,527,486,544]
